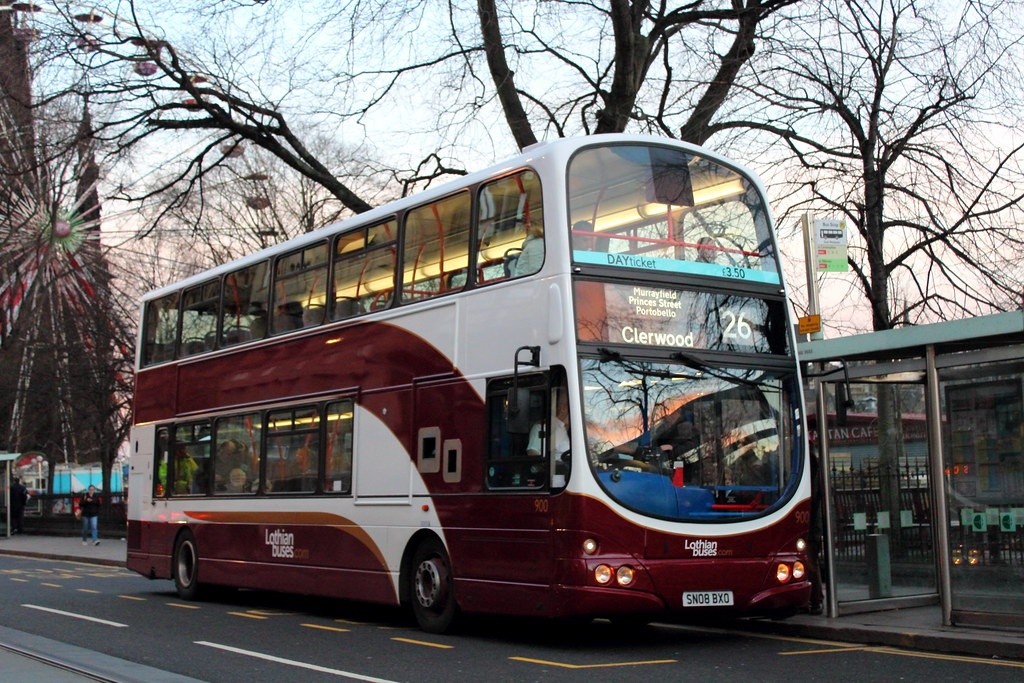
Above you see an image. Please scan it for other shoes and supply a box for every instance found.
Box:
[92,541,100,546]
[82,539,88,546]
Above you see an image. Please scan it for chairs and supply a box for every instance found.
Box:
[151,248,688,497]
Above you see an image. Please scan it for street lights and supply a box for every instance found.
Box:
[31,456,49,513]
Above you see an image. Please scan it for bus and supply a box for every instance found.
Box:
[118,129,817,636]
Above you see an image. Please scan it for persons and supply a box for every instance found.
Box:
[79,485,101,546]
[695,237,720,265]
[651,398,699,486]
[712,466,741,504]
[10,477,27,535]
[526,386,636,488]
[514,224,545,277]
[572,220,594,251]
[154,427,266,498]
